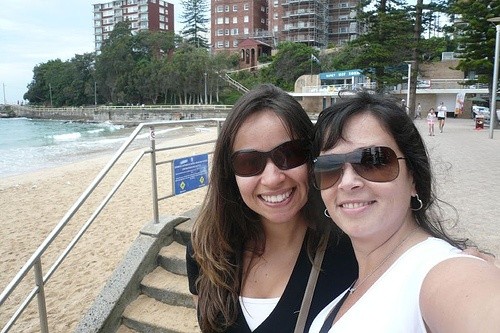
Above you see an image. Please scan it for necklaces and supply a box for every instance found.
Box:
[349,254,392,294]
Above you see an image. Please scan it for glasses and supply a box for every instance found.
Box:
[229,139,310,177]
[310,146,408,190]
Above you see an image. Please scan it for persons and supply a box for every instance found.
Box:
[415,103,423,119]
[186,84,358,333]
[437,102,447,133]
[427,108,436,136]
[310,88,500,333]
[400,98,411,111]
